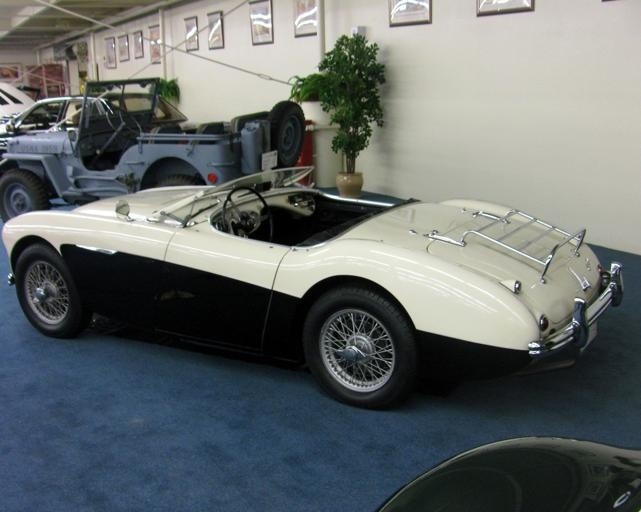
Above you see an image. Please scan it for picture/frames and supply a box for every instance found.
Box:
[117,34,130,62]
[133,30,145,60]
[0,62,24,83]
[104,36,117,69]
[475,1,535,17]
[388,0,433,29]
[206,11,225,51]
[293,0,318,39]
[248,1,275,46]
[183,16,200,51]
[148,24,161,64]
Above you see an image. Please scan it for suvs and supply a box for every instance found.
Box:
[1,78,306,222]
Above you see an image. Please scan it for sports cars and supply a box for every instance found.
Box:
[0,163,625,409]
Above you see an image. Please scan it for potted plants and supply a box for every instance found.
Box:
[289,31,387,199]
